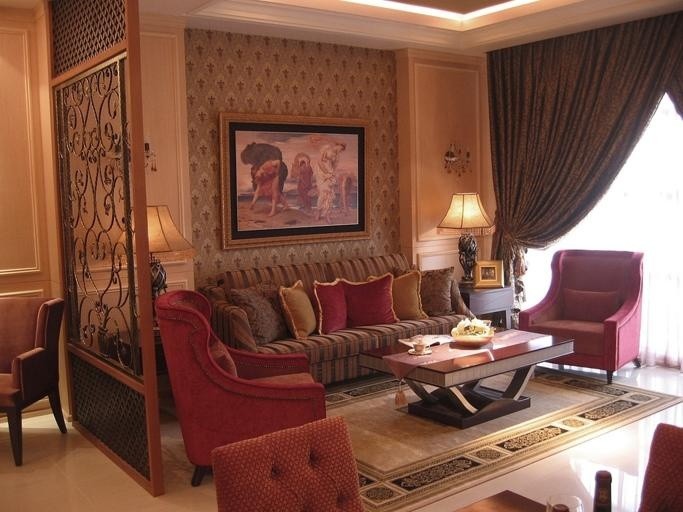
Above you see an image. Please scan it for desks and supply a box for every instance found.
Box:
[106,326,166,380]
[454,485,559,512]
[455,283,519,329]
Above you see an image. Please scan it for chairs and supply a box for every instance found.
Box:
[517,249,646,386]
[150,284,329,489]
[210,412,364,510]
[0,295,65,469]
[636,419,682,511]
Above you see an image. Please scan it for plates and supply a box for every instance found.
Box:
[409,349,430,355]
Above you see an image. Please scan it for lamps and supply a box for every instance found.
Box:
[439,140,473,177]
[117,199,192,330]
[432,191,498,286]
[142,141,158,175]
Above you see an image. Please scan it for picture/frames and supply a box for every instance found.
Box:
[216,108,376,251]
[473,259,506,289]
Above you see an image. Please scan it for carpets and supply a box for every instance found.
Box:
[295,363,681,512]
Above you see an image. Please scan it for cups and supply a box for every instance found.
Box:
[545,492,585,511]
[482,319,491,327]
[413,343,429,352]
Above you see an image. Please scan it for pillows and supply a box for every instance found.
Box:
[227,262,456,349]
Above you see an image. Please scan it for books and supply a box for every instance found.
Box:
[396,334,454,349]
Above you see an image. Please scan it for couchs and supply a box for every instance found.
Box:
[190,250,475,393]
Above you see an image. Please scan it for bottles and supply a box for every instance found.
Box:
[593,469,612,511]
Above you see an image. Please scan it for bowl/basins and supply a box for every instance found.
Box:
[451,331,493,348]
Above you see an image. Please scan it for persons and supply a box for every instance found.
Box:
[295,160,312,211]
[249,159,288,217]
[315,142,344,219]
[337,174,351,211]
